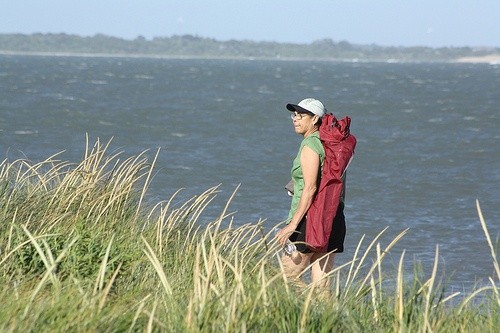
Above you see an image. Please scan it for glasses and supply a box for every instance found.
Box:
[290,113,310,120]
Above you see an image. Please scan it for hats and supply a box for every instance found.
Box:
[286,97,327,118]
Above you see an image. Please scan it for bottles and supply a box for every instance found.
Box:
[273,226,296,254]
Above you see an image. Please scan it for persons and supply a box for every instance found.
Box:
[274,98,346,287]
